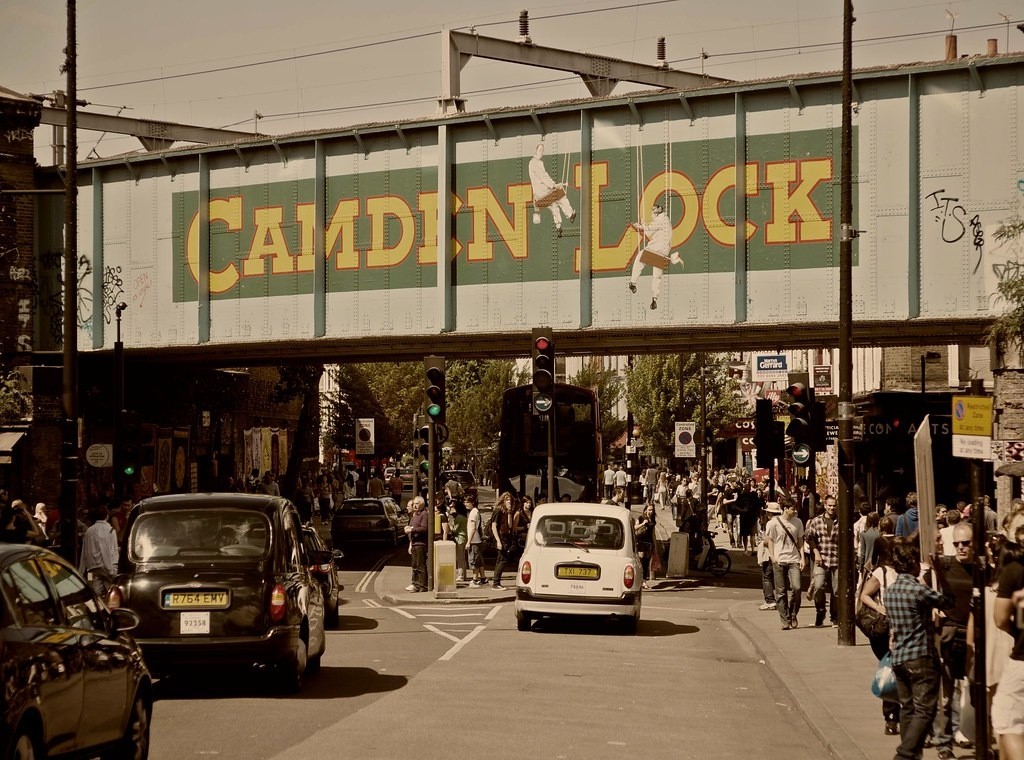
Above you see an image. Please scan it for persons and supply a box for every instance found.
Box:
[223,462,403,525]
[764,497,806,630]
[884,542,956,760]
[0,487,135,605]
[853,493,1024,760]
[601,461,815,611]
[404,474,571,592]
[806,496,839,628]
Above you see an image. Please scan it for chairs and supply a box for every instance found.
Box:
[596,521,614,540]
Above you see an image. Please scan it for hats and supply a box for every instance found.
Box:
[963,503,972,516]
[96,506,111,514]
[761,502,783,513]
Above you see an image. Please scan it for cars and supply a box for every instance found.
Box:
[0,543,155,760]
[301,520,345,631]
[105,491,336,697]
[383,465,429,493]
[514,502,653,636]
[330,494,409,551]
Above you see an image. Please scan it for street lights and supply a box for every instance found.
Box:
[699,360,748,533]
[921,351,942,421]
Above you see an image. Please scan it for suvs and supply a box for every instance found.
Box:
[435,469,479,508]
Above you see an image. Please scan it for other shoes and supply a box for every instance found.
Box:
[953,730,973,748]
[830,619,838,628]
[455,575,465,582]
[411,587,426,593]
[480,577,489,584]
[470,580,482,585]
[937,749,958,760]
[923,740,931,749]
[884,720,898,736]
[814,612,826,627]
[781,622,789,630]
[759,601,777,610]
[790,618,798,628]
[405,584,415,591]
[491,584,507,591]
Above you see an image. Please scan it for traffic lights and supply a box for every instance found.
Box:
[786,371,816,467]
[116,436,140,482]
[424,355,447,424]
[531,327,556,417]
[418,415,430,478]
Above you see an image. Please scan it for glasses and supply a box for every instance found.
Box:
[952,540,972,548]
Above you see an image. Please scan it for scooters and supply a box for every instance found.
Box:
[656,527,731,578]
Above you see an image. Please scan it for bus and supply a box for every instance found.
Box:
[497,382,611,511]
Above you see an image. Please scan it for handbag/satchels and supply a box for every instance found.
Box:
[649,552,662,573]
[853,564,890,640]
[871,650,899,709]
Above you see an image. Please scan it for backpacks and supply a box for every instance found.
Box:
[481,519,496,547]
